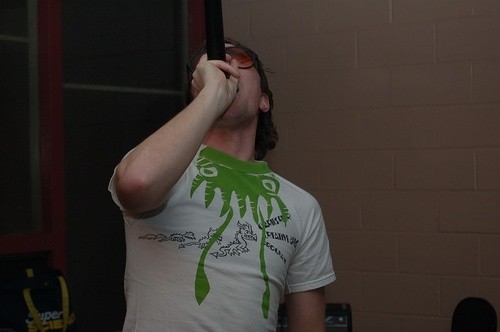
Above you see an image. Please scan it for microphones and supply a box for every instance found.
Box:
[202,0,229,83]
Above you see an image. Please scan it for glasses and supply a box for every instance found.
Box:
[187,47,262,77]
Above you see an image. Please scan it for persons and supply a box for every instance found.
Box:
[107,38,336,332]
[452,297,497,332]
